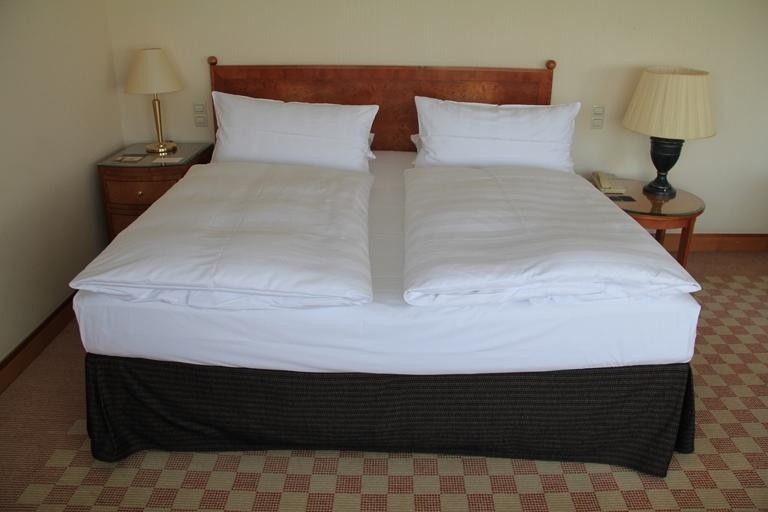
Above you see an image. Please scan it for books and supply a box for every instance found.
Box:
[113,152,147,163]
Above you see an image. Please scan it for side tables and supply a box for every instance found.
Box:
[591,177,705,272]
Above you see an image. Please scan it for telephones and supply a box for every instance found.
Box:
[592,172,628,194]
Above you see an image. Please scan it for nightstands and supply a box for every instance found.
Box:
[97,142,212,244]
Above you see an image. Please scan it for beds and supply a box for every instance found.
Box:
[69,51,704,480]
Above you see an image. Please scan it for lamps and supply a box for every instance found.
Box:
[621,66,718,197]
[125,45,187,156]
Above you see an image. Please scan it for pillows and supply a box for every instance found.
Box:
[205,87,381,171]
[409,92,583,173]
